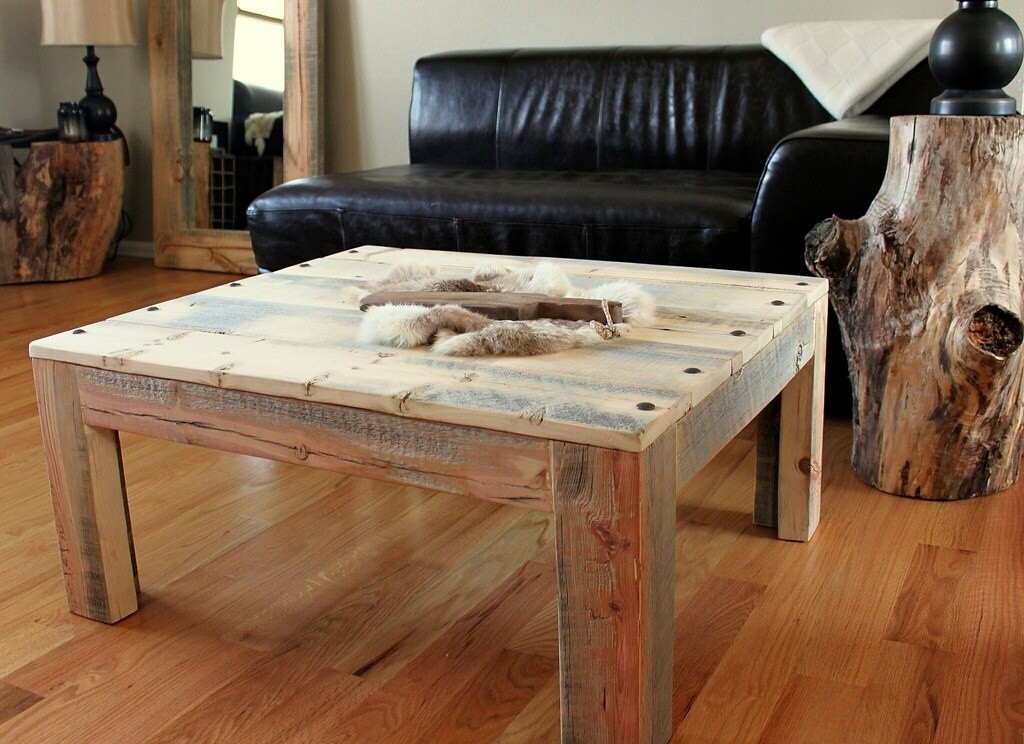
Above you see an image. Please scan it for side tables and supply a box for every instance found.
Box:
[803,114,1023,501]
[0,141,126,286]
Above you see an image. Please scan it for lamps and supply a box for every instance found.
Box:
[39,0,140,141]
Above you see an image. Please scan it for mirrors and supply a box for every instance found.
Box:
[147,1,324,274]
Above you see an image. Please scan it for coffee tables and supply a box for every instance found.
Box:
[27,244,829,744]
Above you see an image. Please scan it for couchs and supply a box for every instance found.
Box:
[231,79,283,230]
[246,43,946,428]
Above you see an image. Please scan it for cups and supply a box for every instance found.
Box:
[57,101,86,143]
[194,106,213,143]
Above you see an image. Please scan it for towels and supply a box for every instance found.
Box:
[760,19,951,121]
